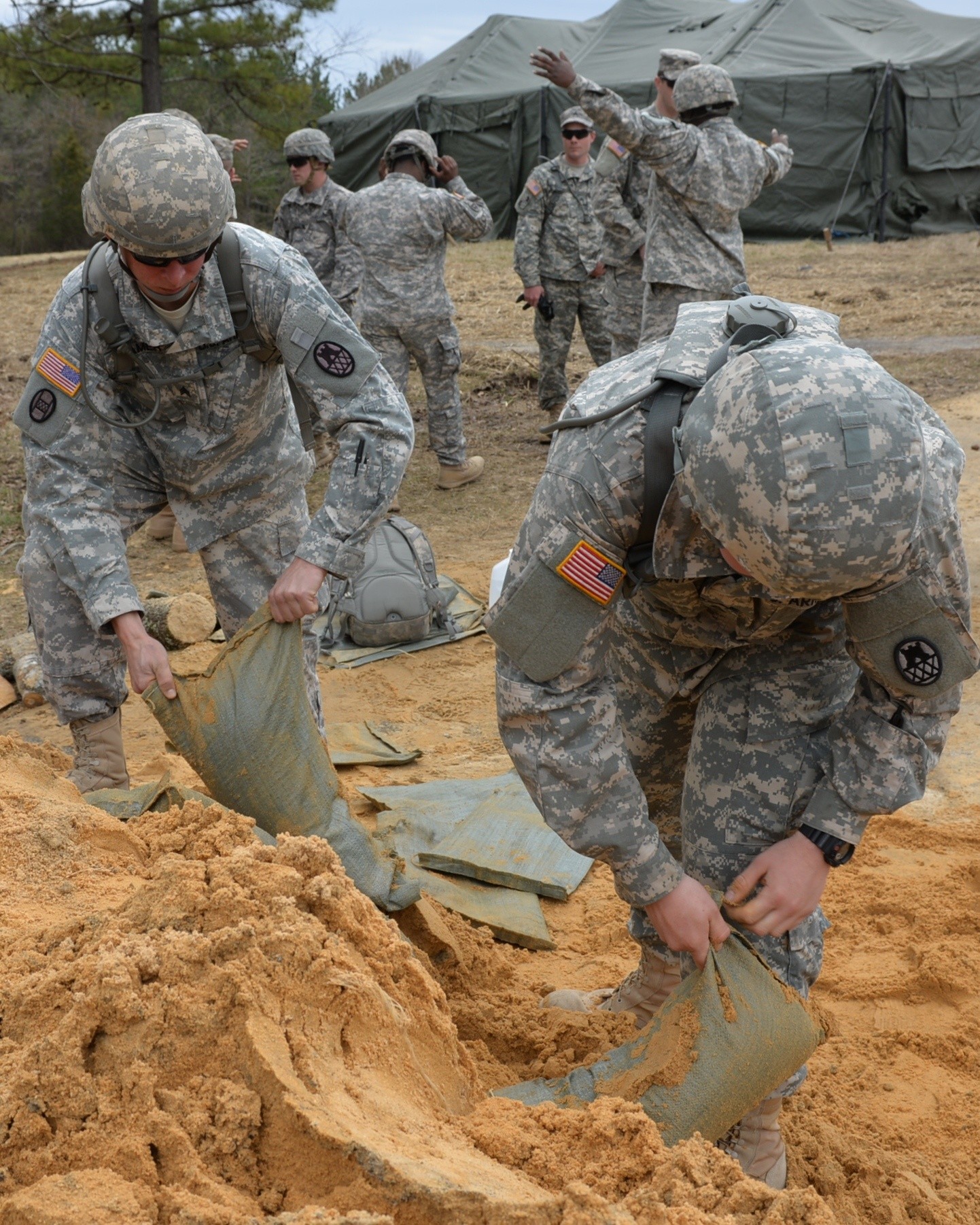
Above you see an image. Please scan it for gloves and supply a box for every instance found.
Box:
[515,294,554,323]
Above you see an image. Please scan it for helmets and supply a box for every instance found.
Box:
[672,64,739,112]
[384,129,438,172]
[284,128,334,163]
[682,341,924,599]
[81,112,235,257]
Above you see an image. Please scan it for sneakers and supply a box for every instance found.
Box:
[438,455,484,489]
[310,436,333,468]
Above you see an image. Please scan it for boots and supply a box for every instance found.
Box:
[717,1097,786,1191]
[66,707,129,792]
[538,944,681,1031]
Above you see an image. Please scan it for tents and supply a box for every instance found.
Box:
[309,0,980,243]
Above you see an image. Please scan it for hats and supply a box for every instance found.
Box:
[658,49,701,77]
[560,106,594,127]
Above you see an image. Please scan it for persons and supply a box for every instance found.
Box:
[485,294,980,1205]
[270,127,364,465]
[17,114,414,790]
[513,105,611,444]
[338,129,494,488]
[590,48,702,359]
[160,107,249,182]
[530,49,793,350]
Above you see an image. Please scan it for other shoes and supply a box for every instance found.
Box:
[549,403,565,425]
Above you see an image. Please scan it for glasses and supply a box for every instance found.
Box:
[130,245,208,267]
[562,128,593,140]
[286,155,313,167]
[662,77,677,88]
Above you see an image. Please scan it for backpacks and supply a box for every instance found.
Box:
[320,513,460,653]
